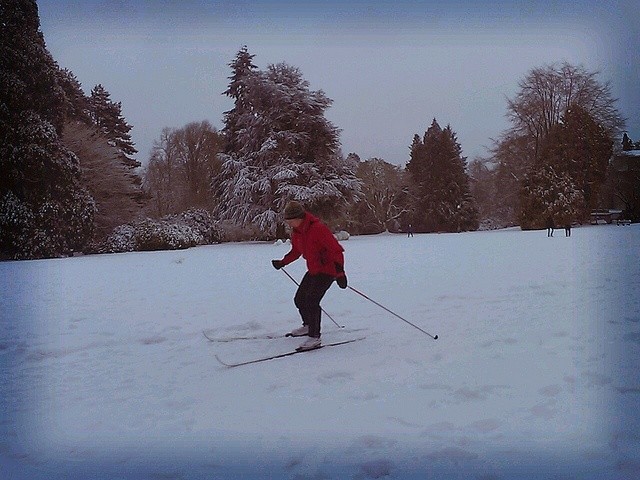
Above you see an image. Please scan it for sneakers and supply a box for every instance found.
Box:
[299,336,322,348]
[291,324,309,336]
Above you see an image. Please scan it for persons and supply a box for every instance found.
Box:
[272,201,346,350]
[407,224,413,237]
[564,217,571,237]
[545,215,554,237]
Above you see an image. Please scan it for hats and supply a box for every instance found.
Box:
[284,201,305,220]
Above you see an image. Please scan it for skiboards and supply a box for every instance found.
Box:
[203,325,368,368]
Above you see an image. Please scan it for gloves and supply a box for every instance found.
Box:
[272,259,284,270]
[336,264,347,289]
